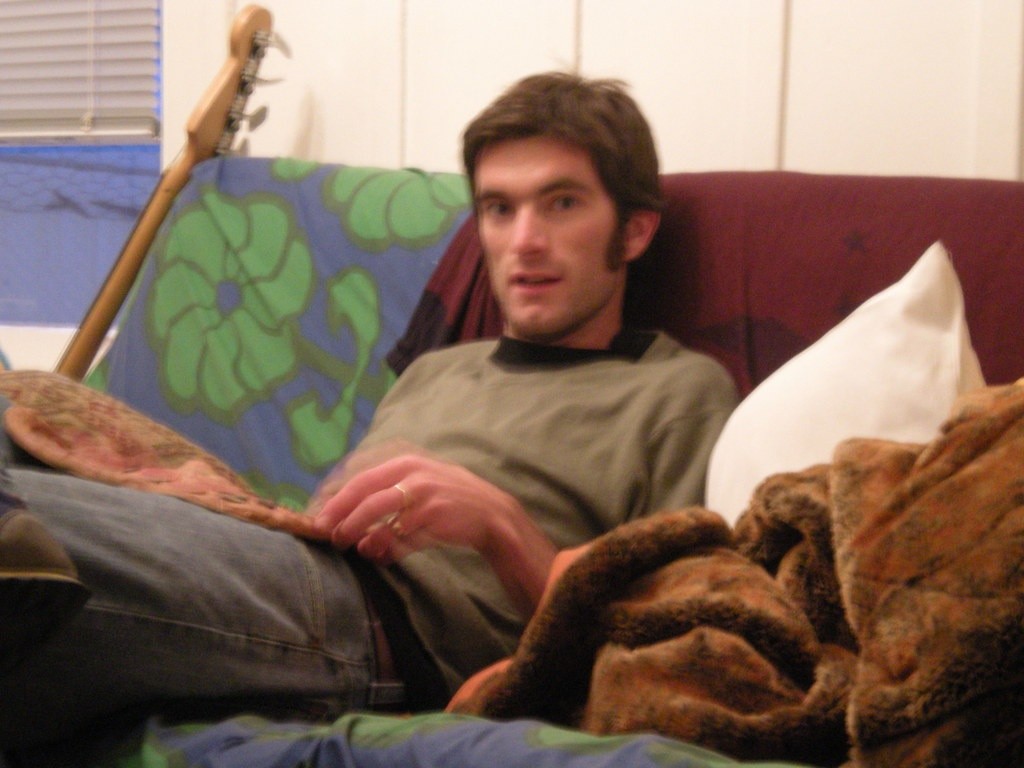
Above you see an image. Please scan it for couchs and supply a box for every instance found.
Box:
[68,154,1022,767]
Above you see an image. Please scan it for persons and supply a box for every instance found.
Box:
[0,71,738,768]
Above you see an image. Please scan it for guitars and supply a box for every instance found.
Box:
[56,4,295,382]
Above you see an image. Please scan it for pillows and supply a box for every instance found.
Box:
[702,239,990,530]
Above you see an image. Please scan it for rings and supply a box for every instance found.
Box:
[395,484,413,505]
[387,516,407,537]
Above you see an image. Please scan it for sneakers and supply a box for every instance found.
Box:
[0,504,90,611]
